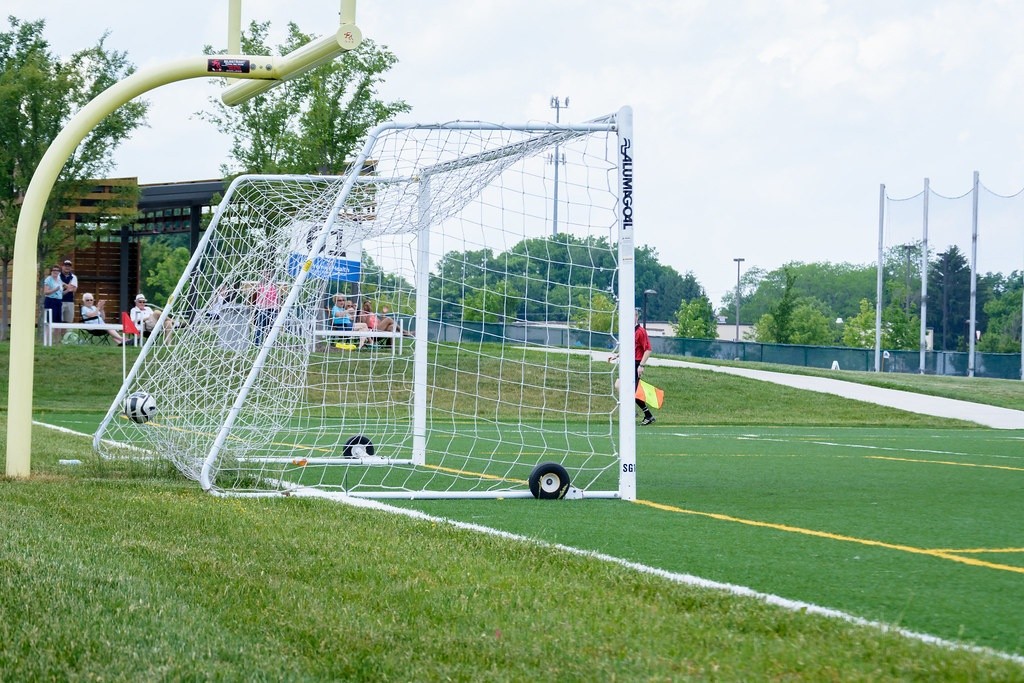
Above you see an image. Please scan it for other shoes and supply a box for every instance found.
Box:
[174,321,182,329]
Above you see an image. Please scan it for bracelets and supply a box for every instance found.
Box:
[639,364,645,367]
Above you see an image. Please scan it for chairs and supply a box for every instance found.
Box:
[78,303,111,345]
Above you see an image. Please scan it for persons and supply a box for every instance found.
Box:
[608,309,656,425]
[44,259,78,347]
[252,269,279,346]
[331,293,416,349]
[81,293,134,347]
[130,294,187,348]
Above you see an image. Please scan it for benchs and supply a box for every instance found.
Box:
[312,319,403,355]
[43,309,144,348]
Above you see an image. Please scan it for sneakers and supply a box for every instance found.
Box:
[638,416,656,426]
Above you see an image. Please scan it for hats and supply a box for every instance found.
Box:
[133,293,148,302]
[63,259,73,266]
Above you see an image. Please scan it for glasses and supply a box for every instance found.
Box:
[337,299,345,301]
[139,301,145,303]
[86,299,93,301]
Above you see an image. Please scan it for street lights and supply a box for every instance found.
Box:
[643,289,657,328]
[963,320,979,352]
[904,246,916,320]
[733,258,744,342]
[937,252,948,351]
[548,97,569,235]
